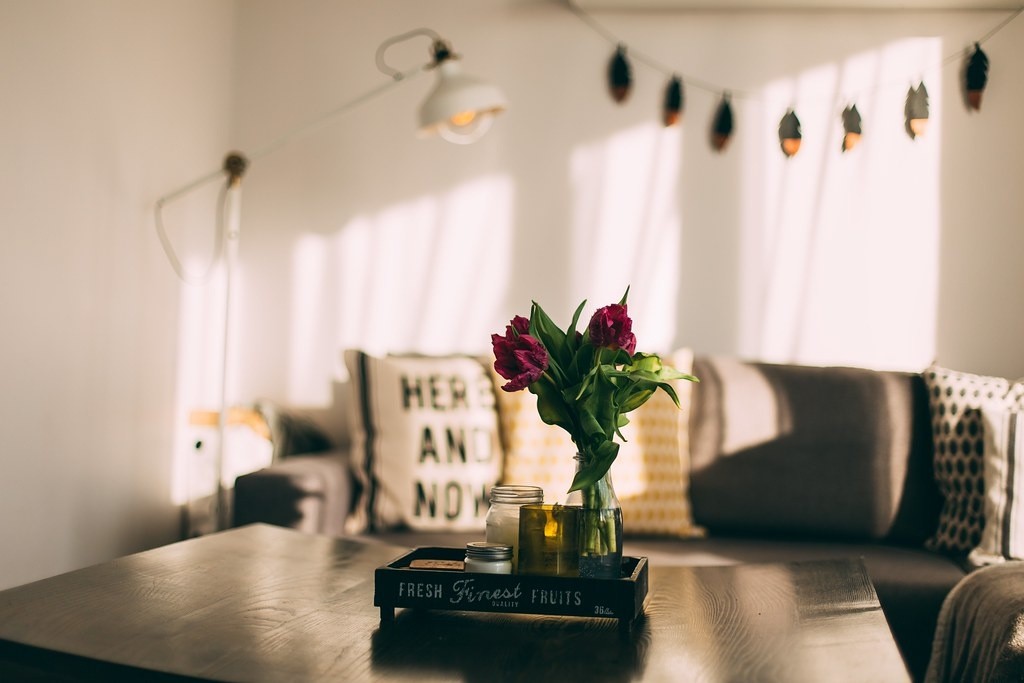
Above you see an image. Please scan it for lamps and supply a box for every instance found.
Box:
[149,29,509,534]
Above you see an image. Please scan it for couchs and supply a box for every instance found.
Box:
[231,351,1023,621]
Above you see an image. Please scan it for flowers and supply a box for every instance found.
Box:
[491,283,703,559]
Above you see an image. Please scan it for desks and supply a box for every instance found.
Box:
[2,520,914,683]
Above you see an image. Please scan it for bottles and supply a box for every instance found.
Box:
[464,541,514,574]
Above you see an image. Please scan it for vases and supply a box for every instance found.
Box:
[516,501,625,579]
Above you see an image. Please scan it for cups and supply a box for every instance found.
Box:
[517,505,579,579]
[485,485,546,575]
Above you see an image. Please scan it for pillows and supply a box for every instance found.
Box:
[915,369,1024,555]
[341,345,503,538]
[685,349,935,547]
[491,344,714,540]
